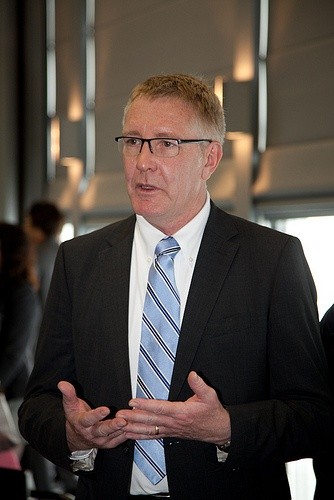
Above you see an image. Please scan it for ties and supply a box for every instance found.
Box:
[129,235,184,486]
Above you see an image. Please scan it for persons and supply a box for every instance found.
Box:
[17,74,324,500]
[313,304,334,500]
[0,200,63,500]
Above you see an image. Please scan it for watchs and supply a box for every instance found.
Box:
[217,441,231,452]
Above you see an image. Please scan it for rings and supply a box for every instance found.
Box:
[155,425,160,435]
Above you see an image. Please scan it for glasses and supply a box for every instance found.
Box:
[115,133,217,157]
